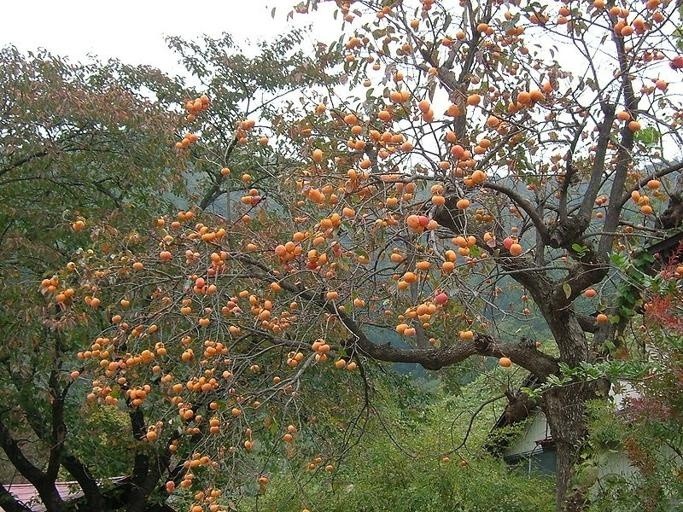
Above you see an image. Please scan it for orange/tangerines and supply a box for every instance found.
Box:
[42,0,682,512]
